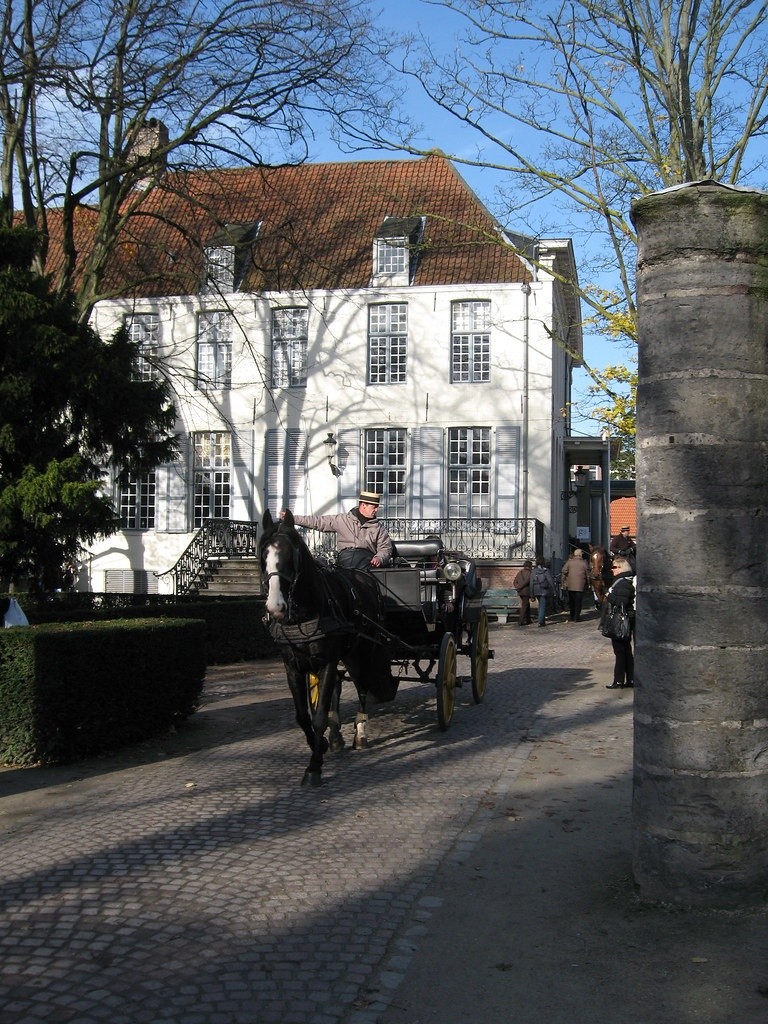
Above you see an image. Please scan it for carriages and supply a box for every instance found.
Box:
[253,508,496,791]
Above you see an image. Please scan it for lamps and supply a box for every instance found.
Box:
[323,433,341,477]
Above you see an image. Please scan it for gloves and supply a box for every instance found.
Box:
[620,551,626,555]
[626,549,631,554]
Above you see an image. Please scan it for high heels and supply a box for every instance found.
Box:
[624,679,634,688]
[606,679,624,689]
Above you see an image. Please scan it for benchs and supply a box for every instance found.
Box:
[381,539,443,585]
[481,589,519,623]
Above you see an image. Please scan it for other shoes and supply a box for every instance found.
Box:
[518,622,525,625]
[527,621,533,624]
[538,623,546,627]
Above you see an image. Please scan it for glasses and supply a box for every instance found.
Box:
[611,565,620,569]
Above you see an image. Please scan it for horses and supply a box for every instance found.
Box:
[257,508,380,788]
[587,542,612,610]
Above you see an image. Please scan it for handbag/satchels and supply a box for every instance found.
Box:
[539,579,553,596]
[601,601,630,638]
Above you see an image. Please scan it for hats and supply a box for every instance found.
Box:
[620,526,630,531]
[357,491,382,504]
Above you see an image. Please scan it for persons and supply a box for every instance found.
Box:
[278,492,392,568]
[561,525,636,689]
[530,557,556,627]
[513,561,532,626]
